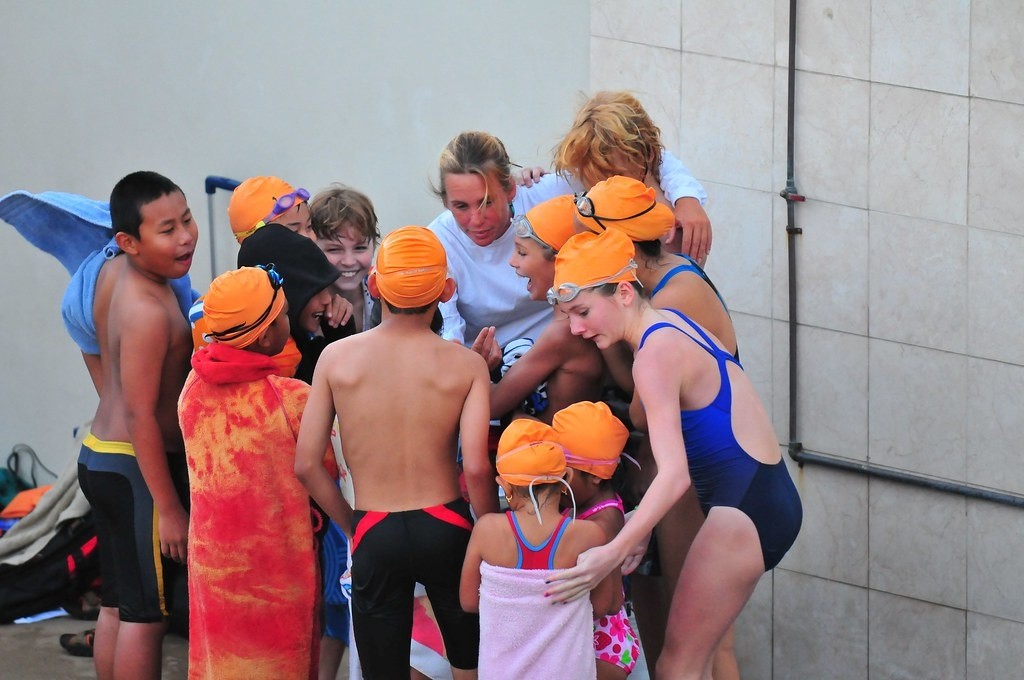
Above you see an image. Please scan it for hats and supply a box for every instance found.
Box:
[375,226,447,308]
[495,418,566,486]
[525,195,595,253]
[575,175,675,242]
[228,176,304,245]
[553,226,637,294]
[552,401,630,480]
[203,266,286,350]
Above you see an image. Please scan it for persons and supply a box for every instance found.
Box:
[549,400,639,680]
[416,131,713,421]
[76,171,199,679]
[233,225,352,680]
[571,175,742,679]
[304,186,388,340]
[292,224,497,680]
[455,417,595,680]
[514,91,688,258]
[546,225,804,679]
[176,263,336,679]
[488,195,606,435]
[228,175,318,252]
[328,302,468,679]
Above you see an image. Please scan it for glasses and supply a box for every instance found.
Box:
[545,283,580,306]
[255,263,285,291]
[273,188,310,215]
[573,190,595,217]
[511,214,532,238]
